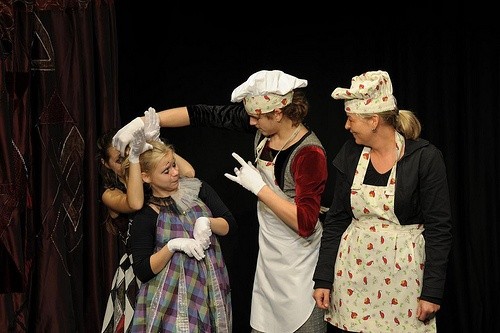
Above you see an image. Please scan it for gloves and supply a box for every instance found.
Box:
[128,127,153,164]
[166,216,213,262]
[112,115,145,156]
[223,152,267,196]
[143,106,164,145]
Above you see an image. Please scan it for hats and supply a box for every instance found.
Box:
[229,69,308,114]
[331,69,398,114]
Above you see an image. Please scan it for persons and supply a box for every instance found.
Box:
[96,129,196,333]
[110,70,328,332]
[313,68,452,333]
[101,141,238,333]
